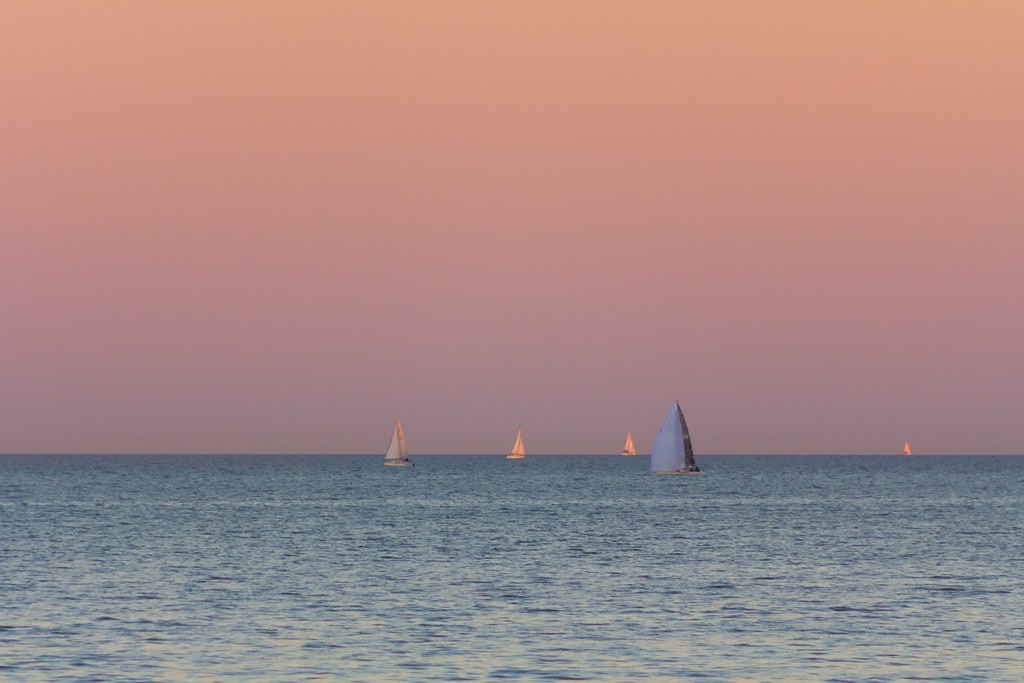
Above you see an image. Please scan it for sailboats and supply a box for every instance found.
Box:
[505,429,526,459]
[619,431,637,457]
[647,401,706,475]
[384,419,414,467]
[903,442,912,456]
